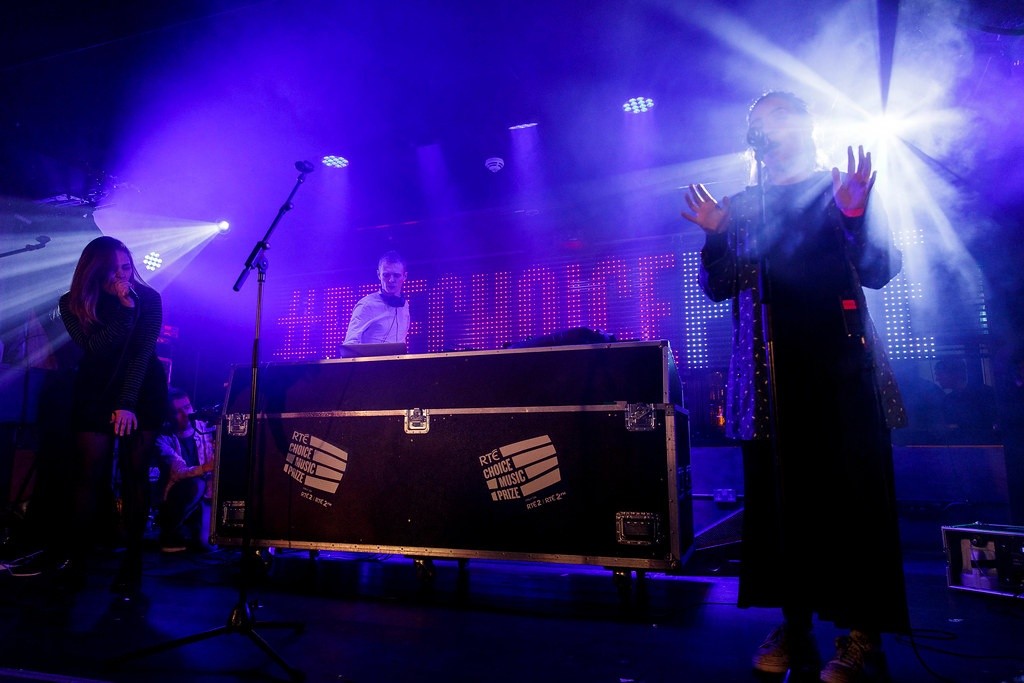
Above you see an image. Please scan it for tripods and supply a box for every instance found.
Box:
[116,160,313,683]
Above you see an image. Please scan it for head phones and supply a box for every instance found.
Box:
[380,289,406,307]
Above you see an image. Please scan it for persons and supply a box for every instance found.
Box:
[680,89,907,683]
[153,386,218,560]
[42,235,169,630]
[342,249,411,345]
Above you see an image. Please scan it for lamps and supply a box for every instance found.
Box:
[320,146,350,167]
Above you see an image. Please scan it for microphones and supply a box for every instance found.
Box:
[747,129,770,149]
[127,285,140,302]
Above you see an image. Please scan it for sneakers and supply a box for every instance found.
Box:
[752,625,814,673]
[820,635,887,683]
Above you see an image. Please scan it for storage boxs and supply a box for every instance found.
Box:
[940,521,1024,599]
